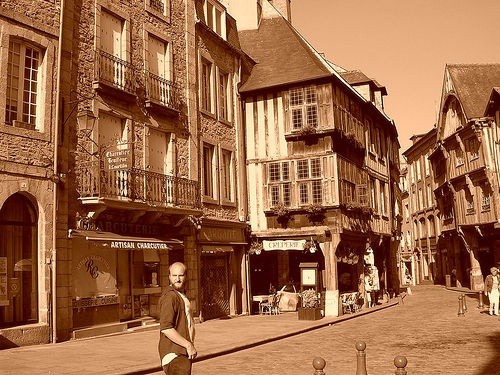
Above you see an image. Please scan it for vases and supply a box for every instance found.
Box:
[255,250,261,255]
[309,247,316,253]
[336,254,359,264]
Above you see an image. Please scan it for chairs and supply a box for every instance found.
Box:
[341,292,363,315]
[260,293,281,316]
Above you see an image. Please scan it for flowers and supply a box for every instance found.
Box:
[335,248,359,259]
[303,241,314,252]
[249,242,263,254]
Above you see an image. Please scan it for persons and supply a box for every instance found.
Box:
[484,267,500,315]
[158,262,197,375]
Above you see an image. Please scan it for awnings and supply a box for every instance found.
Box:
[68,229,184,250]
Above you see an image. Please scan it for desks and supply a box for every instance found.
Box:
[253,295,270,314]
